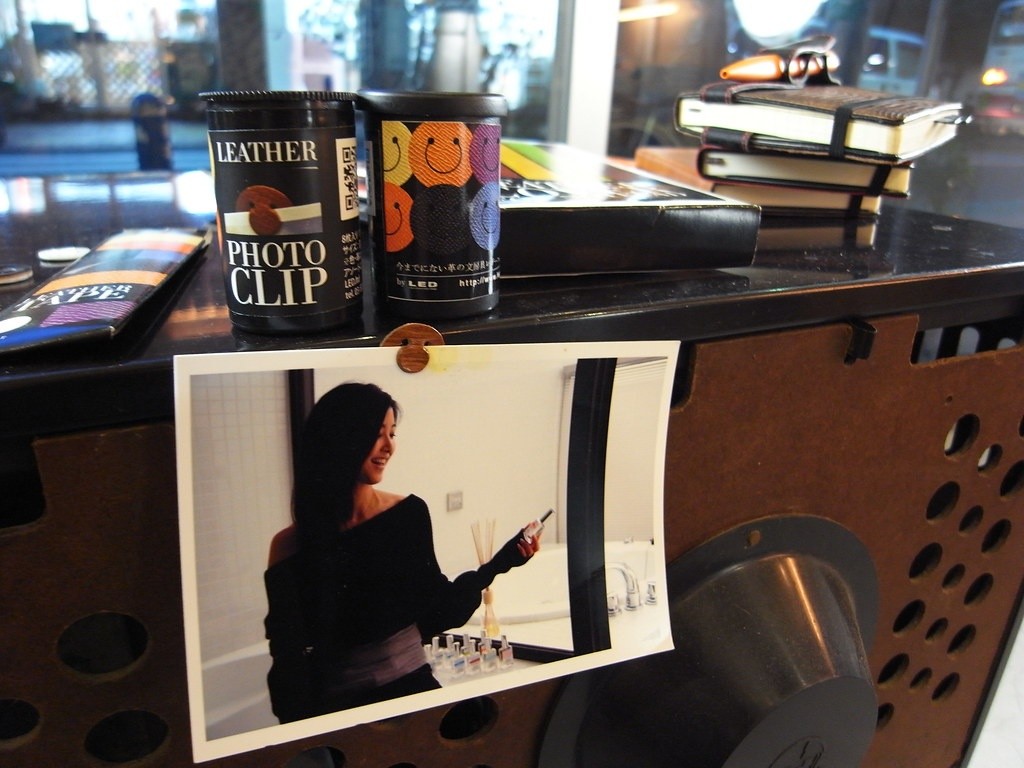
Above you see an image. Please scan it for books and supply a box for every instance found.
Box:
[635,75,967,217]
[496,138,763,275]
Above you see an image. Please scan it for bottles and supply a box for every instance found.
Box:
[461,633,476,657]
[523,507,557,544]
[498,634,514,668]
[467,641,481,675]
[444,635,456,667]
[478,630,492,654]
[423,643,436,673]
[428,635,442,674]
[482,640,497,672]
[450,642,465,677]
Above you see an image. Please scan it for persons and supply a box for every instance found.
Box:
[265,383,541,726]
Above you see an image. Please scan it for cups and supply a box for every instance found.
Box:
[357,89,506,321]
[132,89,176,163]
[195,91,364,331]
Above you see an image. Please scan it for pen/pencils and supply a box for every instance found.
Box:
[720,53,842,79]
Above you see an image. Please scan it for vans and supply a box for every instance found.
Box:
[796,19,939,104]
[975,1,1024,136]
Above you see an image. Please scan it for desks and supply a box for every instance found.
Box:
[0,165,1024,768]
